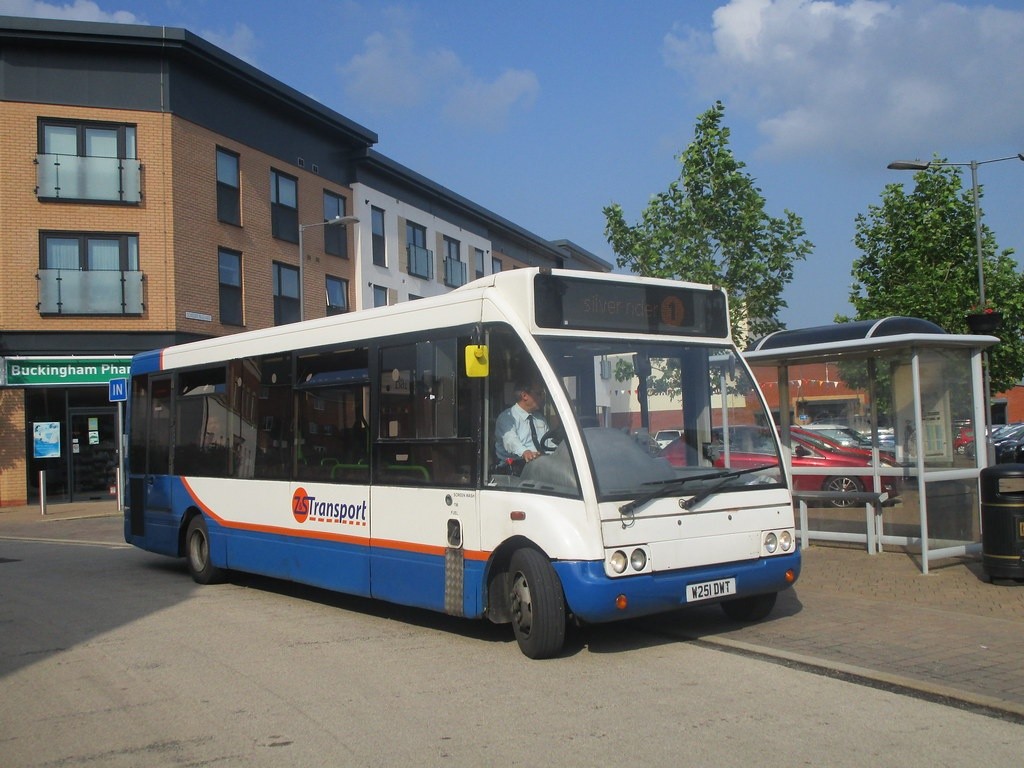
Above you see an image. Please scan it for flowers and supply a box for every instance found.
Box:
[963,304,995,318]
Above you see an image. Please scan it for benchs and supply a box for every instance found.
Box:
[790,490,888,555]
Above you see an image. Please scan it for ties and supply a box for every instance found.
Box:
[527,415,540,451]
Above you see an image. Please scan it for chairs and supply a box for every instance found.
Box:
[297,414,498,485]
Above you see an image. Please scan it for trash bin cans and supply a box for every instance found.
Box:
[979,463,1024,583]
[922,480,973,541]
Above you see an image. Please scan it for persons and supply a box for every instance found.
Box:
[198,412,226,447]
[495,375,557,470]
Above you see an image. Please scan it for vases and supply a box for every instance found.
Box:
[964,311,1002,334]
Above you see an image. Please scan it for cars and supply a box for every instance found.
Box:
[661,426,904,509]
[954,423,1024,464]
[654,430,682,449]
[776,426,896,464]
[796,424,896,458]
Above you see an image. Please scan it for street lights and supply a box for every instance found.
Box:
[299,217,360,321]
[886,153,1024,467]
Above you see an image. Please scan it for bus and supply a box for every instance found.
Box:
[124,268,803,661]
